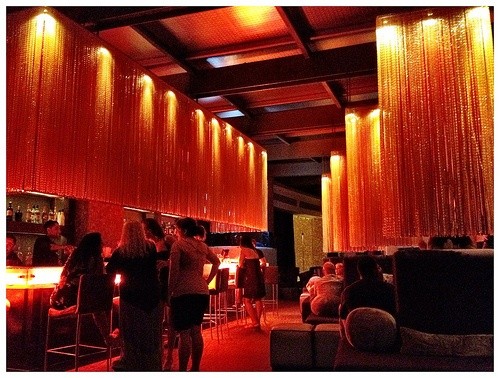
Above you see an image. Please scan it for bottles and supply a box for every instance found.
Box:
[6,202,55,224]
[17,246,33,265]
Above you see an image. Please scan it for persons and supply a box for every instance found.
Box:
[339,256,398,320]
[239,234,268,331]
[314,262,343,296]
[50,232,121,346]
[32,220,61,266]
[105,220,162,372]
[141,218,172,349]
[156,218,220,372]
[6,233,23,266]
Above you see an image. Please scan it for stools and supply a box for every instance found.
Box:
[44,274,114,372]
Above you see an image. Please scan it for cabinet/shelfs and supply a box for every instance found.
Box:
[6,189,75,266]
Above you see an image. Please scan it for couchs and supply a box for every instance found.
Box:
[270,248,494,372]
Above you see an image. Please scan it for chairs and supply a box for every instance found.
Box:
[201,266,279,344]
